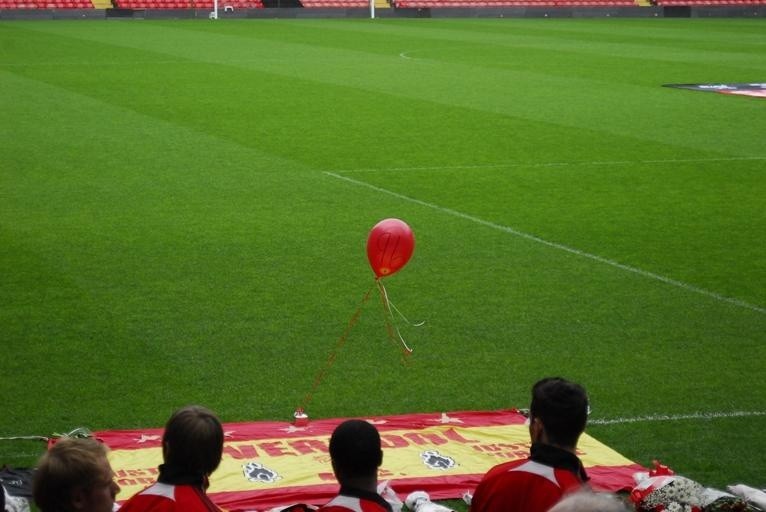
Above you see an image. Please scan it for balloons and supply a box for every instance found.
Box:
[364,217,415,279]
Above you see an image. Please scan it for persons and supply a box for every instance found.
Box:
[31,437,120,512]
[469,378,629,512]
[116,405,229,512]
[317,419,393,512]
